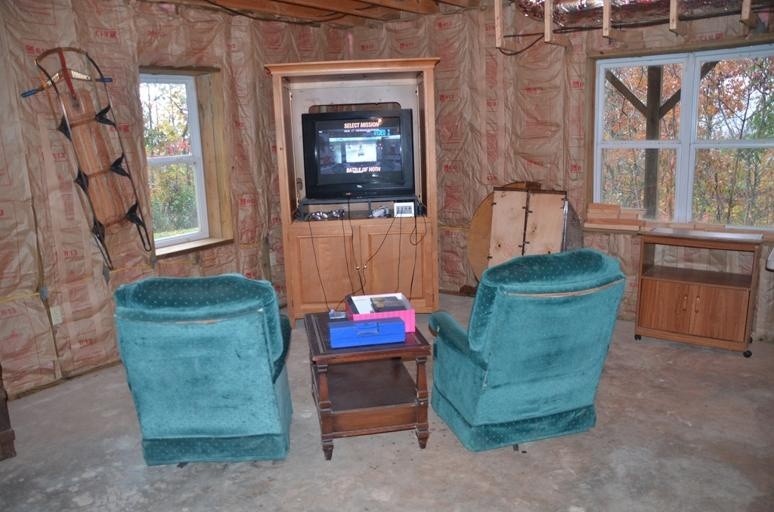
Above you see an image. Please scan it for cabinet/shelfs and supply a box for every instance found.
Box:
[635,232,760,356]
[264,58,439,325]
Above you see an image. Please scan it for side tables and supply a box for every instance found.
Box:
[304,313,430,462]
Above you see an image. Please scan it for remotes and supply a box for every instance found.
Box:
[328,311,346,319]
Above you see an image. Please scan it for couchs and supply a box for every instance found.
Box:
[430,251,626,452]
[116,275,291,465]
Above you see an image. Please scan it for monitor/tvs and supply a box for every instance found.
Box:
[300,109,415,199]
